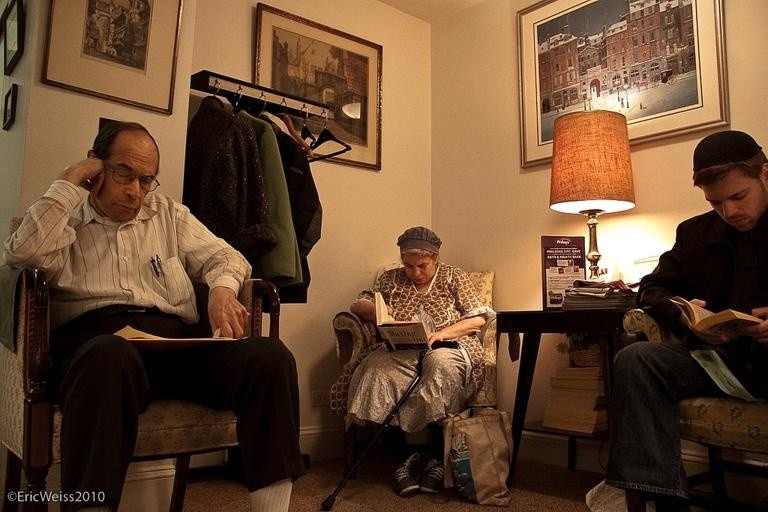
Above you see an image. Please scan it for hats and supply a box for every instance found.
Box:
[397,226,442,255]
[693,131,762,172]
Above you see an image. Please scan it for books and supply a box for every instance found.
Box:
[541,366,610,435]
[560,278,634,312]
[368,292,433,355]
[666,287,765,348]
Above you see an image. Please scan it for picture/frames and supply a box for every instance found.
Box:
[1,0,22,128]
[43,0,184,115]
[515,0,728,174]
[252,0,384,170]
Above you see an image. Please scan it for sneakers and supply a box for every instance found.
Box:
[420,463,445,493]
[394,462,420,495]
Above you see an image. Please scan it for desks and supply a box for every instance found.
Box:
[492,304,640,487]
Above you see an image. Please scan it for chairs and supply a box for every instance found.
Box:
[620,307,767,512]
[332,270,500,483]
[0,212,283,509]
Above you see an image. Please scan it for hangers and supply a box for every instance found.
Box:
[215,79,352,163]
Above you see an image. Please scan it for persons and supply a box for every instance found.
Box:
[605,131,767,511]
[4,122,302,512]
[349,226,492,496]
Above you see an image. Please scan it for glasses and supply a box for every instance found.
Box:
[106,158,160,192]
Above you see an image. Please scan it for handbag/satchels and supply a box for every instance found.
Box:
[440,407,512,507]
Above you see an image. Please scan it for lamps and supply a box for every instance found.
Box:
[546,112,635,304]
